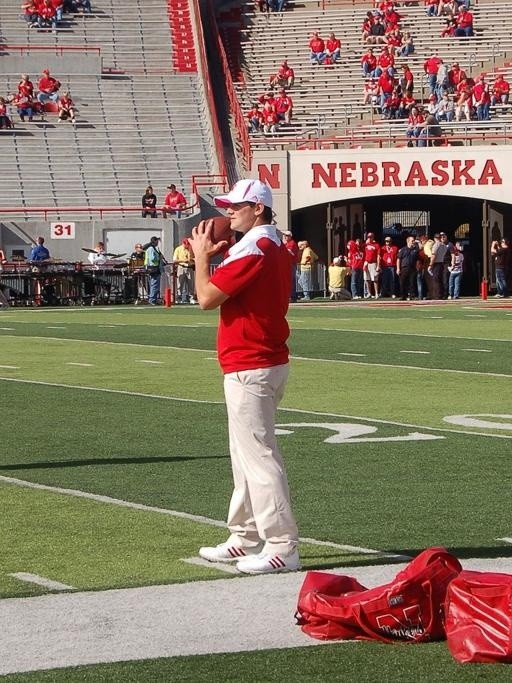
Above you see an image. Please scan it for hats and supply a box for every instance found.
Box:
[249,89,284,110]
[282,229,462,264]
[429,59,502,100]
[380,46,387,50]
[0,69,50,101]
[213,179,272,207]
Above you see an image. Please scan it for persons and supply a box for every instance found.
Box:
[129,244,150,305]
[29,236,49,260]
[0,250,7,263]
[187,178,302,576]
[87,242,108,265]
[21,0,92,33]
[160,183,187,218]
[144,236,164,306]
[0,69,76,130]
[173,237,197,304]
[247,0,510,147]
[142,186,156,218]
[491,238,512,299]
[282,212,478,304]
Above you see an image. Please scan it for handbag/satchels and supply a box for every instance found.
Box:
[145,265,161,275]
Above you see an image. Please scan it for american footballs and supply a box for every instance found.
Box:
[196,215,234,244]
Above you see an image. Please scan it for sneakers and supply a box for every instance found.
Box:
[300,296,310,301]
[72,116,76,124]
[330,292,460,299]
[199,539,263,562]
[58,117,63,122]
[231,549,302,575]
[493,293,503,298]
